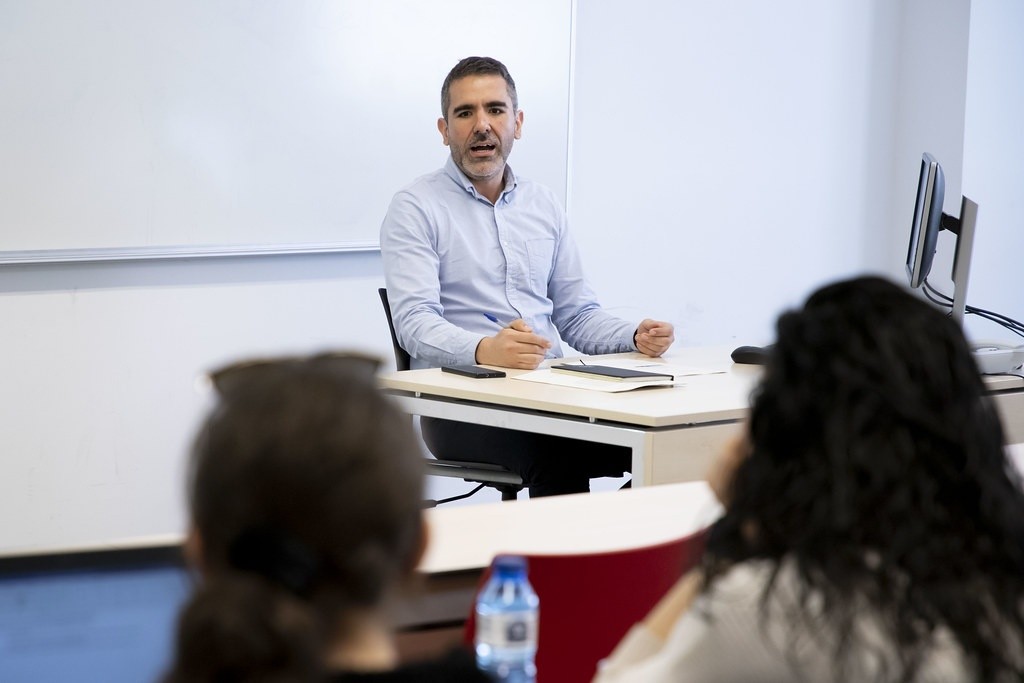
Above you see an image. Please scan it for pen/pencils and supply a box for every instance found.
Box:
[482,311,558,359]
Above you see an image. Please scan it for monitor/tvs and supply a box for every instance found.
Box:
[904,152,945,288]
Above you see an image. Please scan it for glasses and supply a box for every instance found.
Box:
[208,353,381,401]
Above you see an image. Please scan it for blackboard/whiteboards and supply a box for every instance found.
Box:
[0,0,577,268]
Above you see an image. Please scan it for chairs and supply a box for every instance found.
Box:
[380,288,626,497]
[467,511,734,683]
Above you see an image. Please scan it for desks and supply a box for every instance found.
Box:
[365,334,1023,486]
[382,445,1020,658]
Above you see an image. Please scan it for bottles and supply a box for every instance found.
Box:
[474,556,537,681]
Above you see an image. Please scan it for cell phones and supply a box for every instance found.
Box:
[441,364,507,379]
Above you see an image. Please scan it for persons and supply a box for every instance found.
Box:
[591,273,1024,681]
[379,56,674,496]
[148,353,502,683]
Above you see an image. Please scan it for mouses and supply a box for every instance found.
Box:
[731,346,770,365]
